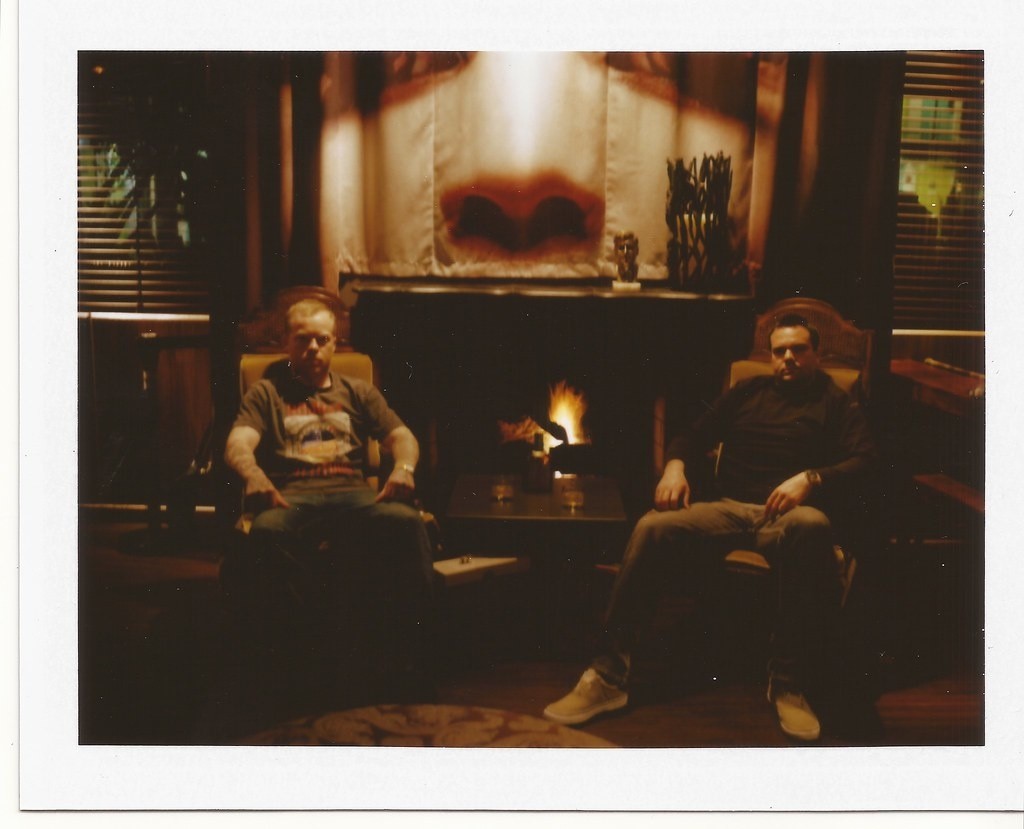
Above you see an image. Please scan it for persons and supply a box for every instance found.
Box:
[615,230,639,282]
[224,301,441,735]
[543,315,888,739]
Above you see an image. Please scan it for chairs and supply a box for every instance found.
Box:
[234,285,440,639]
[713,295,880,617]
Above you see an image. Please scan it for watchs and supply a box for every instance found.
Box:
[807,468,820,488]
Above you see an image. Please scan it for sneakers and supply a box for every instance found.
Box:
[766,678,821,741]
[543,667,629,723]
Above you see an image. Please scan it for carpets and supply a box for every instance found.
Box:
[230,703,629,748]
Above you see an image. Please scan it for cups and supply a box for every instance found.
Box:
[491,475,515,501]
[561,479,586,507]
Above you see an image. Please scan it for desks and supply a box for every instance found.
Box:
[443,475,627,656]
